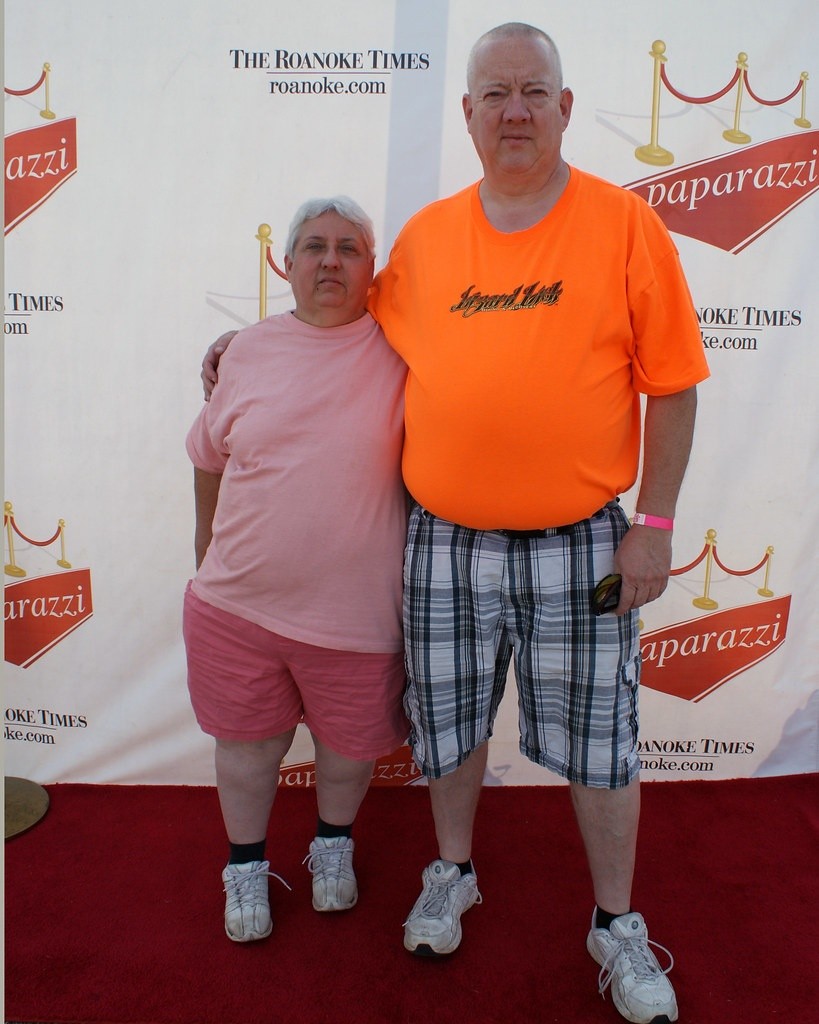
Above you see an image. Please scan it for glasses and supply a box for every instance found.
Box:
[592,574,622,616]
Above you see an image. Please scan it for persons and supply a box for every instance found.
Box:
[202,22,711,1024]
[180,193,411,940]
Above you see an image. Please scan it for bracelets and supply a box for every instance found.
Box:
[632,513,675,530]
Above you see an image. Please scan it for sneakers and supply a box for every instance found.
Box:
[402,857,483,961]
[208,860,293,944]
[301,836,359,914]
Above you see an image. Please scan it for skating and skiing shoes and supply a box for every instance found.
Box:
[586,906,678,1024]
